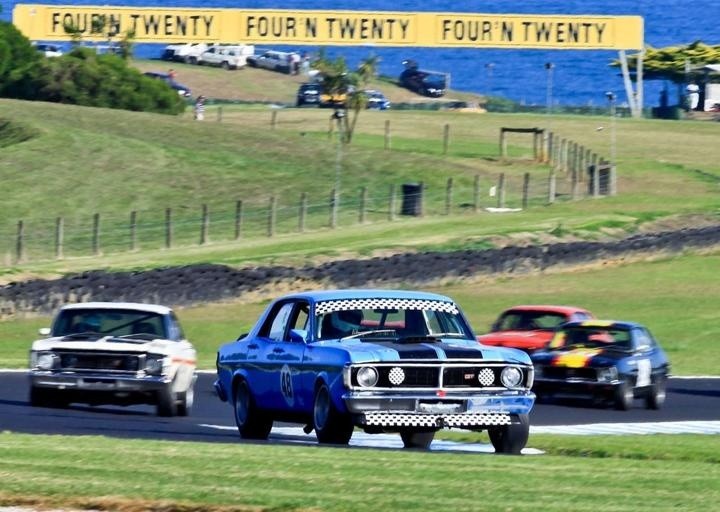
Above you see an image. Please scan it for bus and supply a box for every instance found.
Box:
[401,69,445,97]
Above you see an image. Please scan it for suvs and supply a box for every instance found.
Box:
[164,42,300,75]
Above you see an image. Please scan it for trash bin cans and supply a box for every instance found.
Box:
[402,182,423,215]
[590,164,610,195]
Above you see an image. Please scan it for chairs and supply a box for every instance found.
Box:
[131,322,156,336]
[68,322,100,333]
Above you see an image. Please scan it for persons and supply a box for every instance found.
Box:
[168,67,177,80]
[399,61,444,97]
[317,85,348,141]
[192,94,205,121]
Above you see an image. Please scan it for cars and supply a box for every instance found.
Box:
[297,82,389,110]
[215,288,537,455]
[34,43,63,58]
[28,301,198,415]
[475,307,668,411]
[146,72,192,98]
